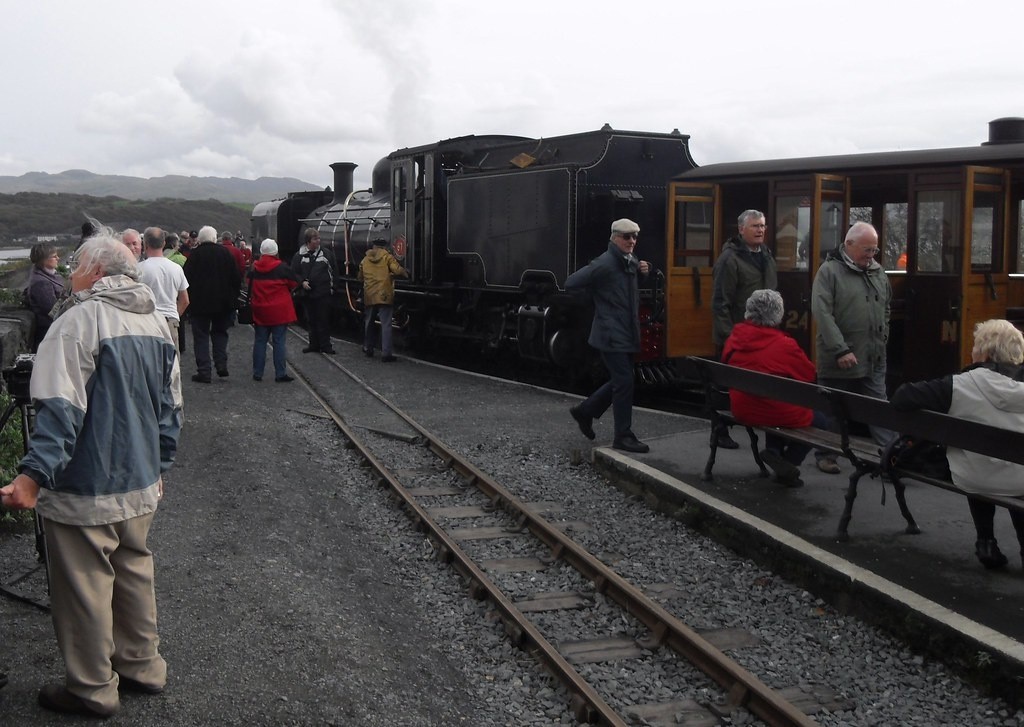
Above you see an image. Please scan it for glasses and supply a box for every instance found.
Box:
[45,254,58,259]
[744,224,768,231]
[615,233,637,241]
[69,260,97,273]
[851,238,880,254]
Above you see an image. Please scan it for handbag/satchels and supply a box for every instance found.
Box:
[880,436,933,471]
[293,281,310,298]
[238,302,254,324]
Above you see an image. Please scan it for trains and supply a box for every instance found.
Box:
[248,116,1024,409]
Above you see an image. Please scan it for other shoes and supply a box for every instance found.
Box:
[38,683,109,719]
[320,345,335,354]
[362,345,374,356]
[769,459,803,489]
[275,374,294,382]
[192,374,211,382]
[612,431,649,453]
[217,368,229,377]
[759,448,777,470]
[710,433,739,450]
[974,537,1009,568]
[118,672,163,695]
[253,374,262,381]
[382,355,397,363]
[816,457,841,474]
[570,401,595,440]
[303,346,320,353]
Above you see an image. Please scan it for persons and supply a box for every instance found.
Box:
[889,319,1024,567]
[292,227,340,354]
[808,221,900,481]
[708,209,785,448]
[720,289,815,486]
[0,212,185,719]
[27,219,251,382]
[358,238,410,362]
[562,218,651,452]
[245,238,303,383]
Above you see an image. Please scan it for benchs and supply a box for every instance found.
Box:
[686,356,1024,545]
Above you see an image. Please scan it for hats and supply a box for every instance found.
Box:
[77,213,281,258]
[372,236,388,246]
[611,218,640,233]
